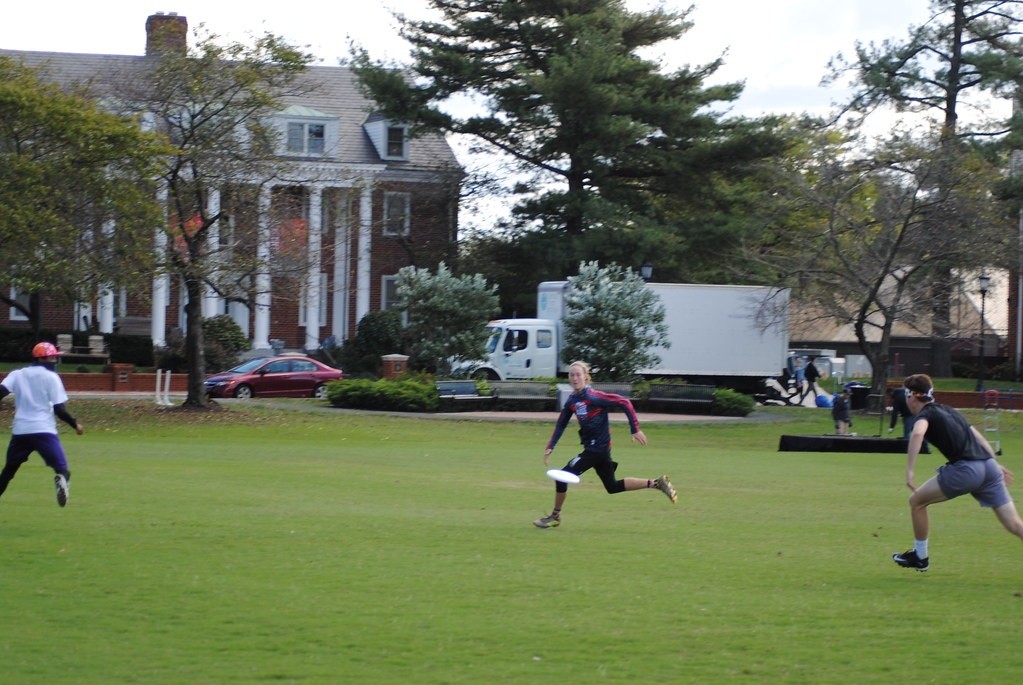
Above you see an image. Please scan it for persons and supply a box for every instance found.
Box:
[886,374,1023,572]
[832,389,853,435]
[533,361,678,529]
[786,357,821,406]
[0,342,84,508]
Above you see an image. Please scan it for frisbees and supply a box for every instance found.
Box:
[545,469,580,484]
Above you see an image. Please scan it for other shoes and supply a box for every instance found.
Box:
[55,474,69,507]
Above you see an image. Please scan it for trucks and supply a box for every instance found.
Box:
[451,281,789,393]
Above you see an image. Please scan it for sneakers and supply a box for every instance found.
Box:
[533,511,560,528]
[893,549,930,572]
[654,475,678,504]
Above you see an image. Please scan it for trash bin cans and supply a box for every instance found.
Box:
[868,394,884,411]
[850,385,872,409]
[555,383,592,412]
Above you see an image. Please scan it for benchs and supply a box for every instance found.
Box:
[589,381,717,417]
[435,380,557,412]
[59,346,112,367]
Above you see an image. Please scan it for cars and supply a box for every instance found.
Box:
[203,355,343,399]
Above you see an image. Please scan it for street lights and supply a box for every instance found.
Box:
[977,269,991,390]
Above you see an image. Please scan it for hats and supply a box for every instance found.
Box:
[843,388,853,394]
[32,342,64,358]
